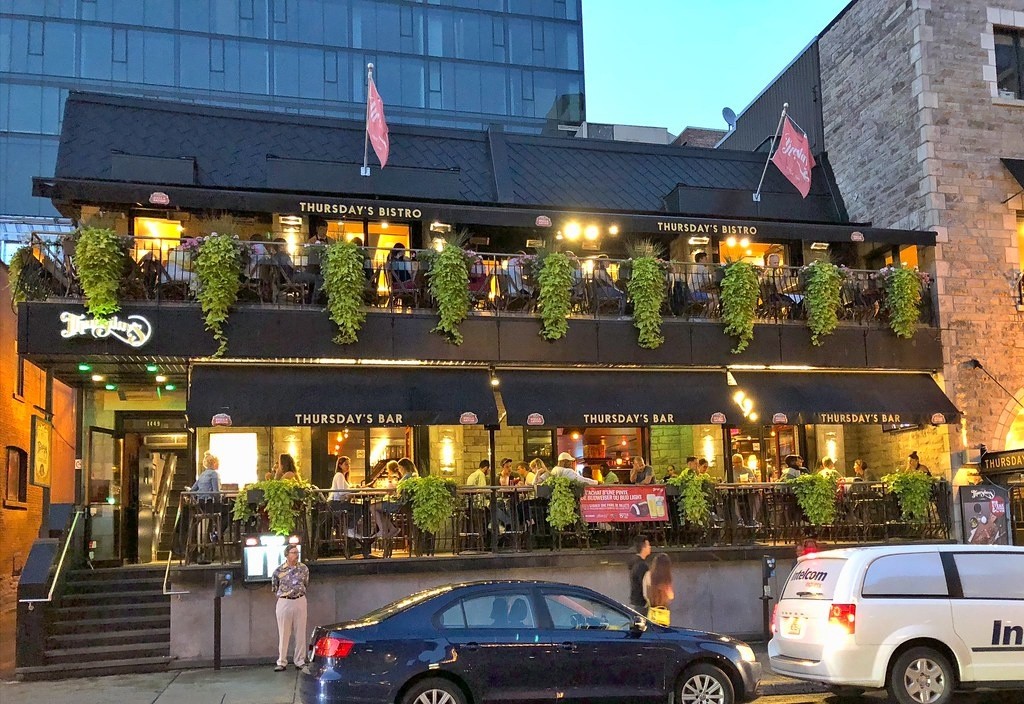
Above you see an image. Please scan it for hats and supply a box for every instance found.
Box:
[558,452,575,461]
[500,458,513,467]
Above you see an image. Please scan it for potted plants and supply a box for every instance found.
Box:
[666,467,729,539]
[520,231,578,341]
[230,476,331,536]
[383,474,470,537]
[788,472,848,526]
[880,467,935,530]
[619,234,671,349]
[536,476,590,530]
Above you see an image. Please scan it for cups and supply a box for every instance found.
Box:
[510,480,514,486]
[740,475,748,483]
[614,478,619,484]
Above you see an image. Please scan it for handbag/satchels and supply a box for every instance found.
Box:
[647,605,670,625]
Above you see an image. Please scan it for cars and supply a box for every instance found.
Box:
[295,577,764,703]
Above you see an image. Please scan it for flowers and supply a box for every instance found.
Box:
[868,261,932,340]
[56,217,137,330]
[416,225,484,347]
[174,206,259,358]
[798,260,855,347]
[715,246,762,356]
[303,214,373,345]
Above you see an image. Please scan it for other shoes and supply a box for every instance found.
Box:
[274,666,286,671]
[713,515,724,521]
[754,519,762,526]
[488,523,506,531]
[297,664,305,669]
[210,532,218,542]
[738,519,744,525]
[343,529,360,539]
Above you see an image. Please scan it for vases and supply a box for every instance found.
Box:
[420,260,430,270]
[716,268,731,282]
[799,273,814,292]
[307,252,323,264]
[867,276,889,290]
[619,267,633,279]
[523,263,545,275]
[192,251,253,265]
[62,240,135,257]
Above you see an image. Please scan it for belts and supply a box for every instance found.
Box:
[281,595,304,599]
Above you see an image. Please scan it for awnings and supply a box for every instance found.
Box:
[186,363,961,425]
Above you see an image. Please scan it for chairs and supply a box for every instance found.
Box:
[591,276,623,315]
[682,283,715,317]
[488,598,528,628]
[929,476,947,539]
[662,280,676,316]
[571,270,589,315]
[759,276,795,319]
[183,496,227,566]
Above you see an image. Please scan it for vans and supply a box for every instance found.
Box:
[766,543,1024,704]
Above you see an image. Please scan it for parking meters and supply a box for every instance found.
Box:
[758,554,777,652]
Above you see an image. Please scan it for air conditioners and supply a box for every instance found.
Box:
[962,449,981,465]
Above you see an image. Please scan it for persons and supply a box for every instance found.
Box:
[904,450,937,520]
[501,249,585,313]
[185,452,233,561]
[791,540,821,571]
[265,454,302,487]
[74,220,376,306]
[593,253,790,315]
[272,544,309,671]
[466,452,620,546]
[799,260,899,321]
[327,456,363,538]
[369,458,419,538]
[624,455,722,545]
[387,243,490,309]
[779,454,877,525]
[724,454,763,527]
[627,535,651,617]
[642,553,674,627]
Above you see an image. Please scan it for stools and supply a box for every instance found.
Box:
[853,499,888,540]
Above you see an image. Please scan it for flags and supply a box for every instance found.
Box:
[368,75,389,170]
[772,116,817,199]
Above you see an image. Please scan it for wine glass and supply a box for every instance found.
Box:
[514,478,518,486]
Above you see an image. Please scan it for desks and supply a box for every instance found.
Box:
[350,491,388,559]
[496,487,534,554]
[455,487,494,554]
[713,485,772,547]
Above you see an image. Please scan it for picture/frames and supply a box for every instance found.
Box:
[29,414,52,488]
